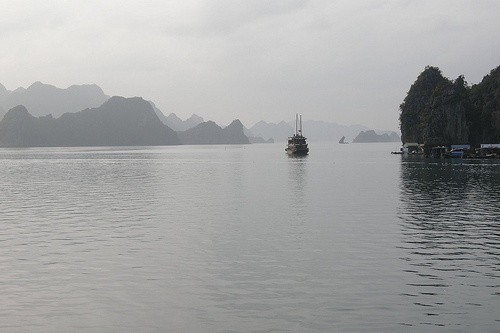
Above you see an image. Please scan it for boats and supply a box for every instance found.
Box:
[285,114,309,154]
[391,142,499,164]
